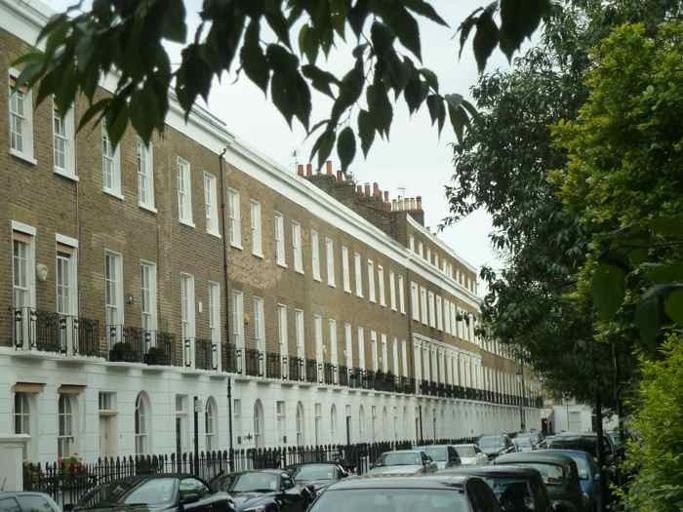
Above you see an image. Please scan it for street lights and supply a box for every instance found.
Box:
[345,405,351,446]
[193,396,204,475]
[610,339,627,487]
[515,369,525,433]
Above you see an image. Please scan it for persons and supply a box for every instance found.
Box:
[600,449,616,509]
[614,454,623,487]
